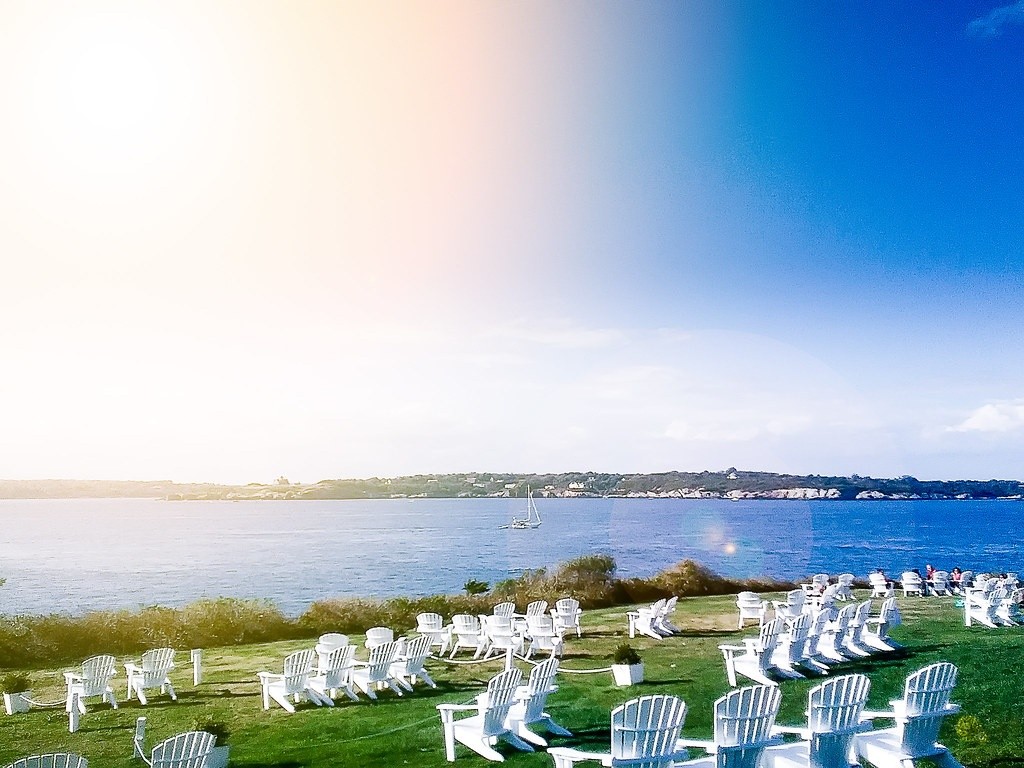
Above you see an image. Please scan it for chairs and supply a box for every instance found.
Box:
[0,571,1024,768]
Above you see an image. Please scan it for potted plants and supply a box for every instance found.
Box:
[192,720,232,768]
[611,643,644,686]
[0,669,36,715]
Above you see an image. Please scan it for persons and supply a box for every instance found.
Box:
[999,574,1008,581]
[925,564,937,580]
[875,569,895,589]
[950,567,961,588]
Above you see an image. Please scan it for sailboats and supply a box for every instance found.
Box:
[497,485,542,529]
